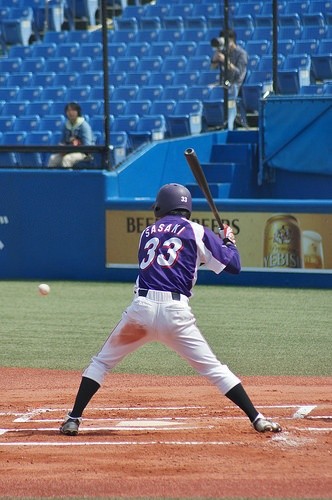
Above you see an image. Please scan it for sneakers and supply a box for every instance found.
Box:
[60,418,79,435]
[254,419,282,433]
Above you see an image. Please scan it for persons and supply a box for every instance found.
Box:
[46,102,94,167]
[210,28,248,93]
[62,183,283,435]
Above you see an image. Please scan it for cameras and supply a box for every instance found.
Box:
[211,37,225,48]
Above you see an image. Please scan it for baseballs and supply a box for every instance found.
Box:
[39,284,49,295]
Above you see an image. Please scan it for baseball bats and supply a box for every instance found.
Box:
[184,148,224,230]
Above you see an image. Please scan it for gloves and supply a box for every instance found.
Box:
[218,224,236,245]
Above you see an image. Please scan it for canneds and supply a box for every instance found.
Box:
[262,214,301,268]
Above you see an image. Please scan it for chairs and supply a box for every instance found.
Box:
[0,0,332,170]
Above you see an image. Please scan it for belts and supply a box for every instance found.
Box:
[138,288,180,301]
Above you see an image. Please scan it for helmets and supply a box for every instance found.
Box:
[154,184,191,219]
